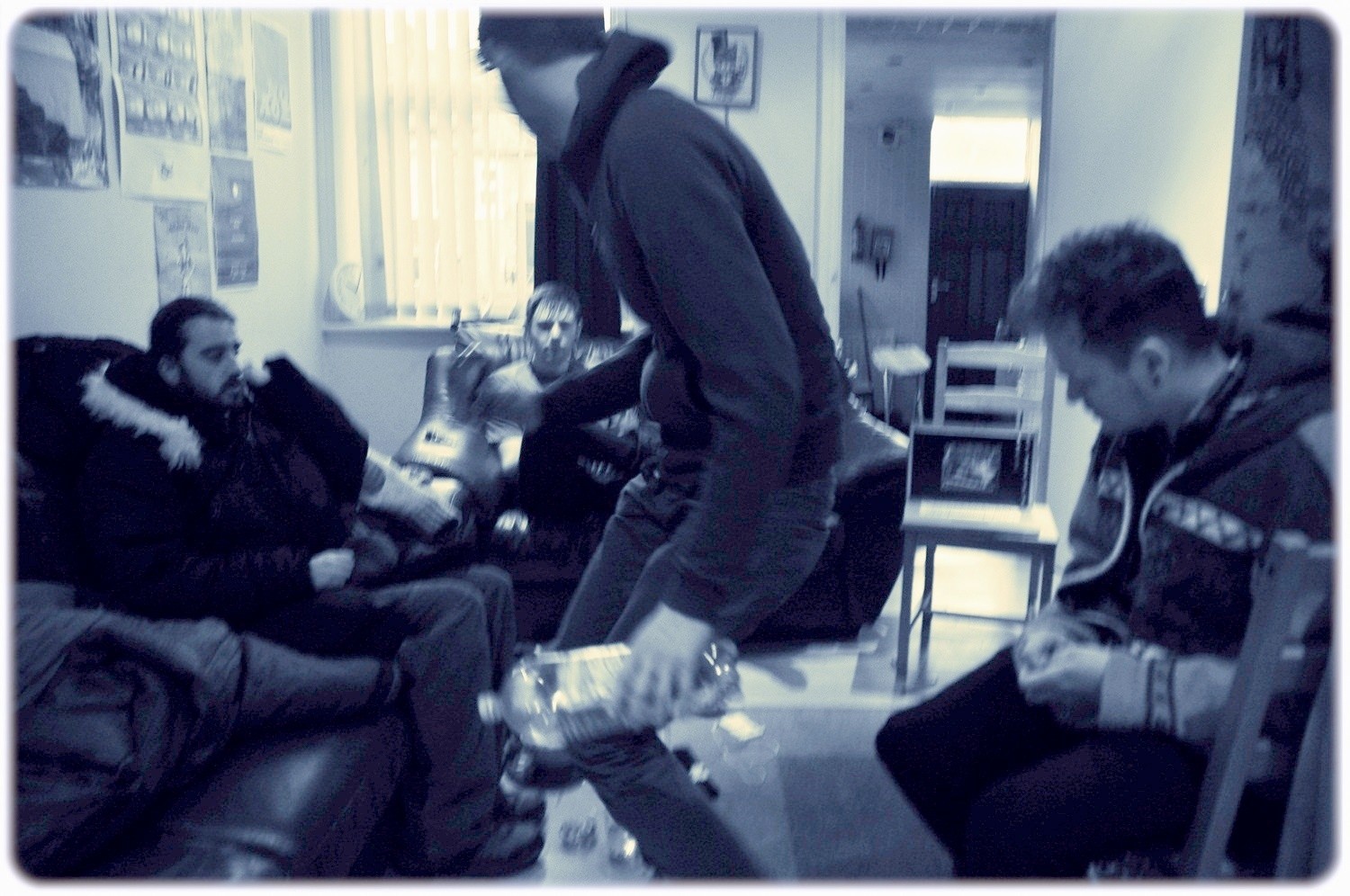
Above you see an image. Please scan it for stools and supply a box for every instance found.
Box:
[872,343,933,442]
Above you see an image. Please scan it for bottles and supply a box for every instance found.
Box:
[476,633,745,752]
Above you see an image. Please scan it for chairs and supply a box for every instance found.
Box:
[1084,533,1336,882]
[896,335,1060,699]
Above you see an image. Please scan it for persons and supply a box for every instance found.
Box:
[876,220,1333,882]
[74,10,851,884]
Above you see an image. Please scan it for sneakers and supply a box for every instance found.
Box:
[497,790,547,821]
[423,821,544,874]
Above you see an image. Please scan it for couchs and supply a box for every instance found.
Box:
[395,318,910,655]
[13,335,416,878]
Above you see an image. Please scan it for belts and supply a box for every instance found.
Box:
[777,463,838,492]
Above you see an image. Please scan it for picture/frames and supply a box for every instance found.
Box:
[695,26,759,111]
